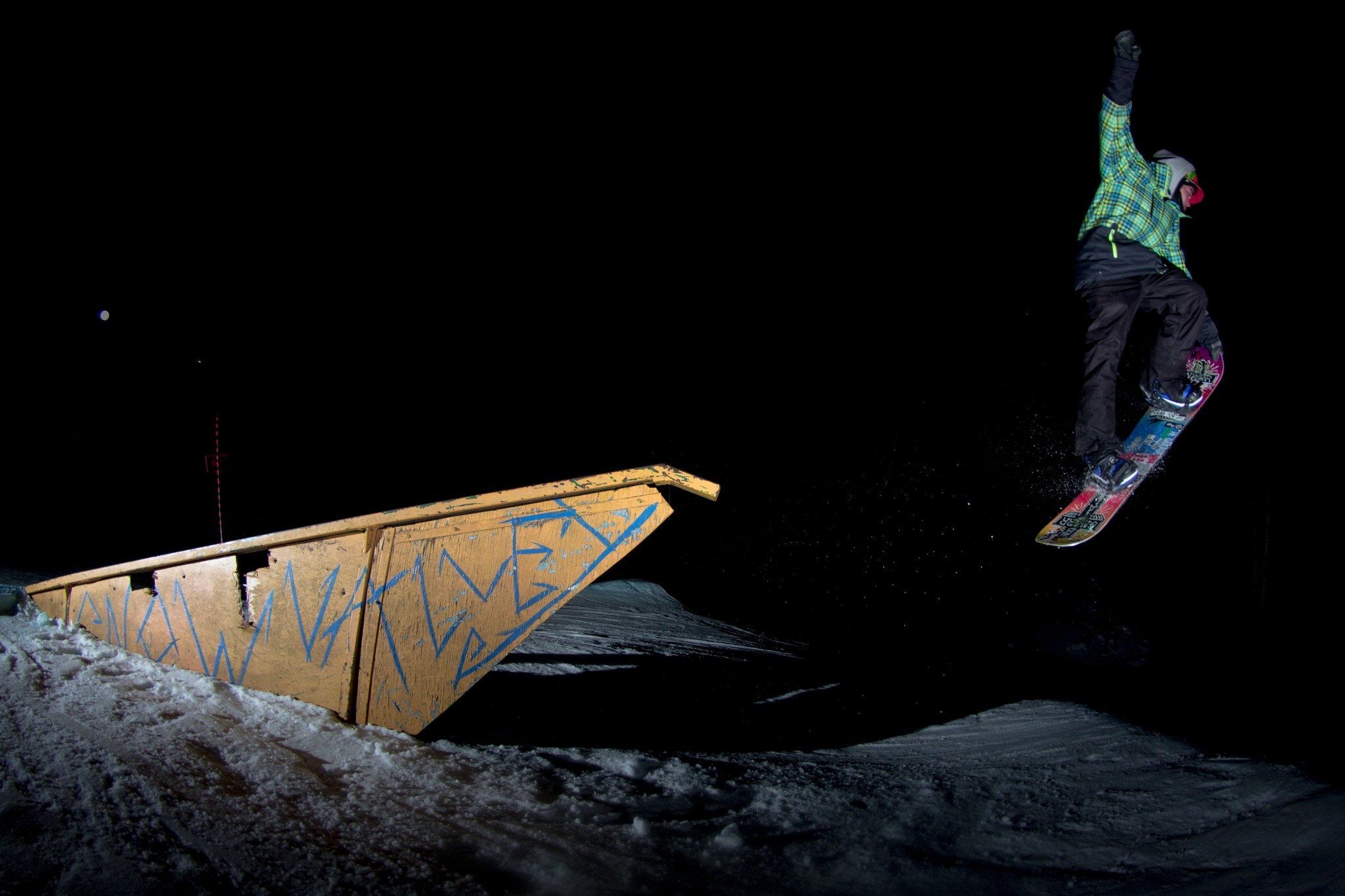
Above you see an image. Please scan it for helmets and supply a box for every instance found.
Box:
[1183,169,1204,204]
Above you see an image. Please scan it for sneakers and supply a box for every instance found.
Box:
[1142,376,1203,408]
[1079,448,1139,490]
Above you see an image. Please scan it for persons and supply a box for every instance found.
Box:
[1068,23,1216,496]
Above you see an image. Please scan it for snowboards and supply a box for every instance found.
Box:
[1035,344,1223,548]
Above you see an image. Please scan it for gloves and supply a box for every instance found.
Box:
[1113,30,1142,61]
[1211,347,1224,361]
